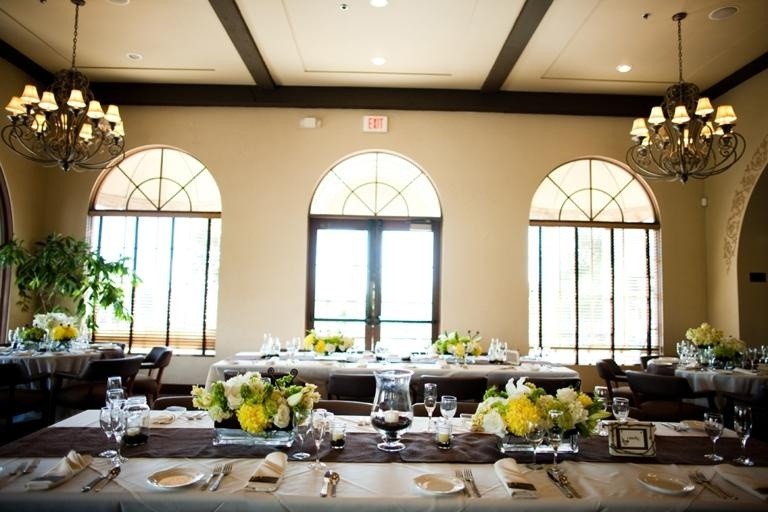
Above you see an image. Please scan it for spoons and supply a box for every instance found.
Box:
[331,472,339,497]
[559,474,582,499]
[96,467,121,492]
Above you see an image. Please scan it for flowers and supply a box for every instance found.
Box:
[305,328,354,352]
[684,324,725,350]
[430,330,482,358]
[45,319,81,351]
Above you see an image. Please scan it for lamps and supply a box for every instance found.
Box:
[626,12,747,184]
[1,1,126,173]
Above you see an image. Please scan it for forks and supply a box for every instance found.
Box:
[463,468,482,498]
[456,470,473,498]
[201,463,223,490]
[210,463,232,491]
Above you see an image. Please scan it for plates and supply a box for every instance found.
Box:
[681,418,702,432]
[146,469,204,489]
[413,473,465,496]
[636,471,695,496]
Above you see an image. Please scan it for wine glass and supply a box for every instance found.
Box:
[286,336,301,356]
[9,329,16,349]
[704,349,717,372]
[17,326,25,350]
[422,383,437,433]
[732,404,754,467]
[499,340,508,362]
[746,347,757,372]
[594,386,608,436]
[525,418,546,470]
[100,376,128,466]
[309,407,326,470]
[292,408,311,459]
[703,410,726,463]
[546,410,565,473]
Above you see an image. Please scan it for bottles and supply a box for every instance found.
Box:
[274,338,281,353]
[123,396,151,446]
[488,338,498,363]
[371,368,414,452]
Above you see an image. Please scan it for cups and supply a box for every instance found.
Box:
[439,395,458,419]
[677,340,696,368]
[330,422,346,450]
[759,346,767,363]
[612,396,629,422]
[264,334,273,354]
[435,421,454,450]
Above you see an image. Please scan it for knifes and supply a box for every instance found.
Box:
[546,470,572,499]
[320,470,333,496]
